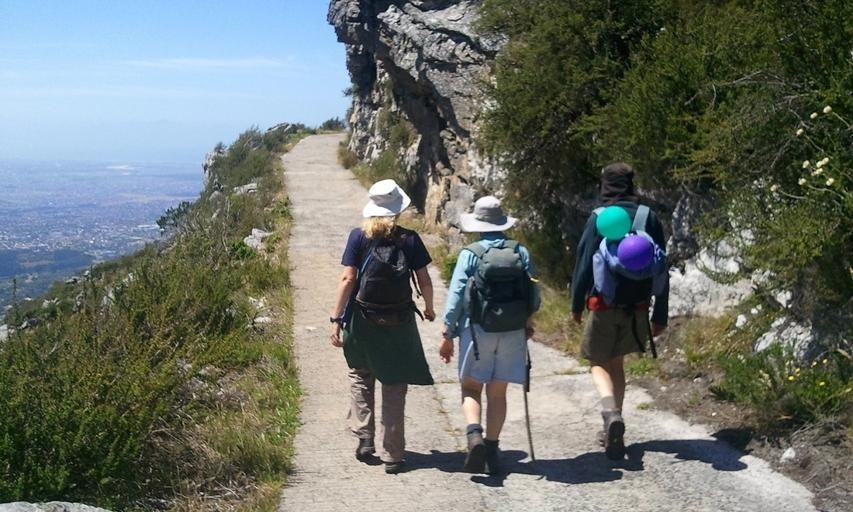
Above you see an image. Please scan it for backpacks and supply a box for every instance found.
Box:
[591,204,667,304]
[463,240,540,331]
[354,227,418,326]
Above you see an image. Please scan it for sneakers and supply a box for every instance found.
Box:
[465,432,497,474]
[386,463,401,474]
[598,411,625,460]
[356,438,375,455]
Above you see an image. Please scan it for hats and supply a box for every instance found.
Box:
[459,196,518,232]
[363,179,411,216]
[600,163,634,199]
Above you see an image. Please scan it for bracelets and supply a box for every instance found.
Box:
[329,316,341,323]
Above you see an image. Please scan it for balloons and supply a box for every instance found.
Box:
[616,235,654,272]
[595,205,631,241]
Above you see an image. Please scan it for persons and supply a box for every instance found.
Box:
[437,195,541,472]
[327,179,435,475]
[569,162,670,463]
